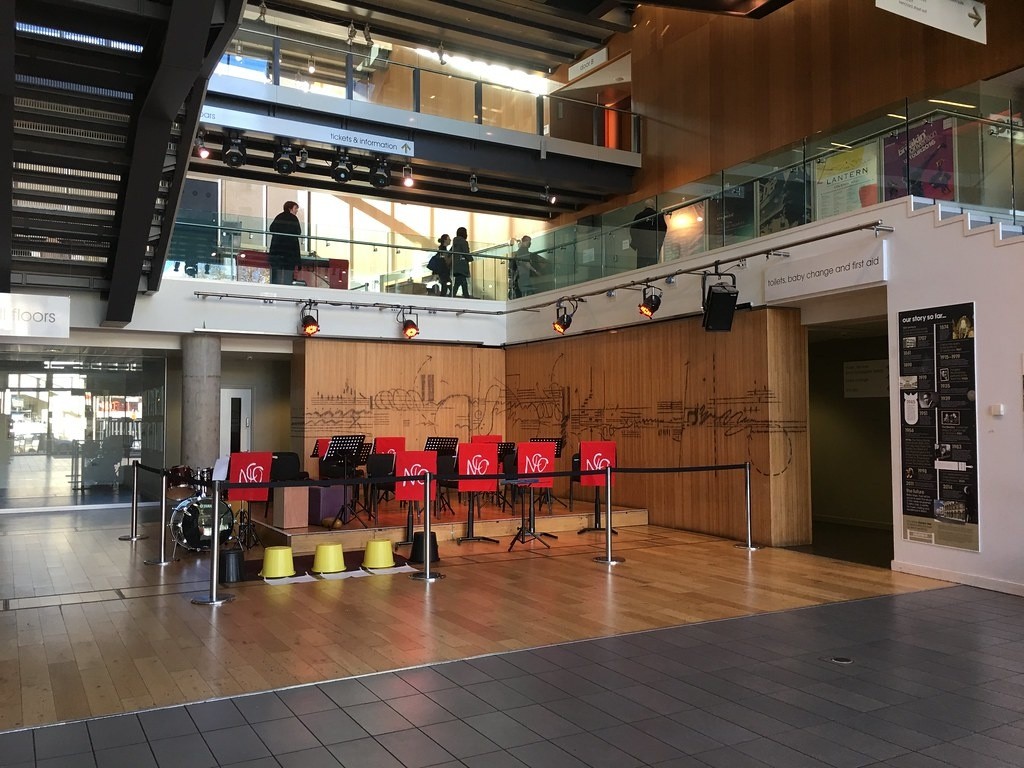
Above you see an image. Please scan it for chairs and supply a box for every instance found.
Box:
[265,448,580,524]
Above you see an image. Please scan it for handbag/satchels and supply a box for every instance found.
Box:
[426,254,446,274]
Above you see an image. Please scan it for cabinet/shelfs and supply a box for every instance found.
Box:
[272,486,309,529]
[309,485,351,525]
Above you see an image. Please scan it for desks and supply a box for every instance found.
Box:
[236,251,349,290]
[385,283,427,294]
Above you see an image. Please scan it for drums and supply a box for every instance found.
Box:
[170,496,235,552]
[194,468,225,499]
[166,465,197,501]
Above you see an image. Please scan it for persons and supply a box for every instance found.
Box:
[515,236,538,297]
[427,227,474,298]
[269,201,302,285]
[630,198,667,269]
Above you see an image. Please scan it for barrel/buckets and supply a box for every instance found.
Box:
[219,547,242,583]
[260,545,297,578]
[409,531,440,563]
[361,538,396,568]
[311,542,347,573]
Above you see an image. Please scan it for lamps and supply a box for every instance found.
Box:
[369,162,392,189]
[540,184,556,204]
[469,174,478,193]
[362,26,374,47]
[403,166,414,187]
[552,298,578,336]
[346,23,357,45]
[273,144,296,178]
[221,136,247,169]
[301,308,320,336]
[639,282,663,318]
[332,157,353,185]
[257,3,267,23]
[437,49,447,65]
[195,139,209,159]
[402,313,420,339]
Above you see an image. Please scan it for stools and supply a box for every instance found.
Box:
[218,549,248,583]
[409,531,440,563]
[259,545,296,578]
[311,543,347,573]
[361,538,396,569]
